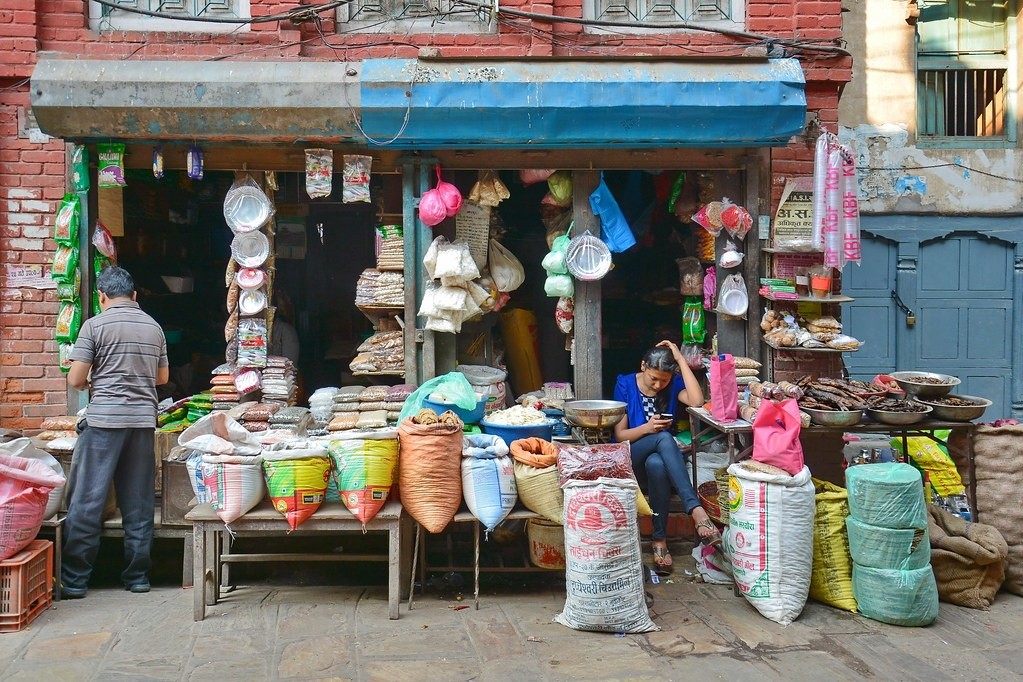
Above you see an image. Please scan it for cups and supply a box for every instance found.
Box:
[812,139,862,269]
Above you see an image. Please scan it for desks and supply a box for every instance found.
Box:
[183,505,403,621]
[686,406,980,598]
[454,508,543,610]
[104,507,194,588]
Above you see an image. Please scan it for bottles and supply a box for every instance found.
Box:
[850,447,910,466]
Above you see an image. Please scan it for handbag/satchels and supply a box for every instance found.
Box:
[415,165,574,333]
[710,354,738,424]
[752,397,804,476]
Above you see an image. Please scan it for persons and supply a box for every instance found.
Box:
[50,263,169,598]
[614,337,723,577]
[272,318,300,369]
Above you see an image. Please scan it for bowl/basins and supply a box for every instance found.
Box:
[888,371,962,396]
[885,392,907,400]
[912,394,994,422]
[422,394,489,424]
[163,328,183,345]
[799,405,864,426]
[868,405,934,424]
[479,418,559,448]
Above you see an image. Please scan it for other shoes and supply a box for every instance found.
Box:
[62,586,87,598]
[130,583,151,592]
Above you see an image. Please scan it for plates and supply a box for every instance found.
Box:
[223,186,272,314]
[722,289,749,316]
[566,236,612,281]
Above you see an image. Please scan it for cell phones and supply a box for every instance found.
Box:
[659,414,673,420]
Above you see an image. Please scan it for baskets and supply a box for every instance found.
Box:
[697,481,730,525]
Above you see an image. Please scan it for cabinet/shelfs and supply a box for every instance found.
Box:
[758,245,860,353]
[347,213,406,377]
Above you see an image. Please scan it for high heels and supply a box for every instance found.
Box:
[695,519,722,547]
[652,546,673,574]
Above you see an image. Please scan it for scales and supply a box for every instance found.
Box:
[551,400,628,444]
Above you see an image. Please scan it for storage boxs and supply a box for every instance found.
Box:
[162,455,196,527]
[0,539,54,633]
